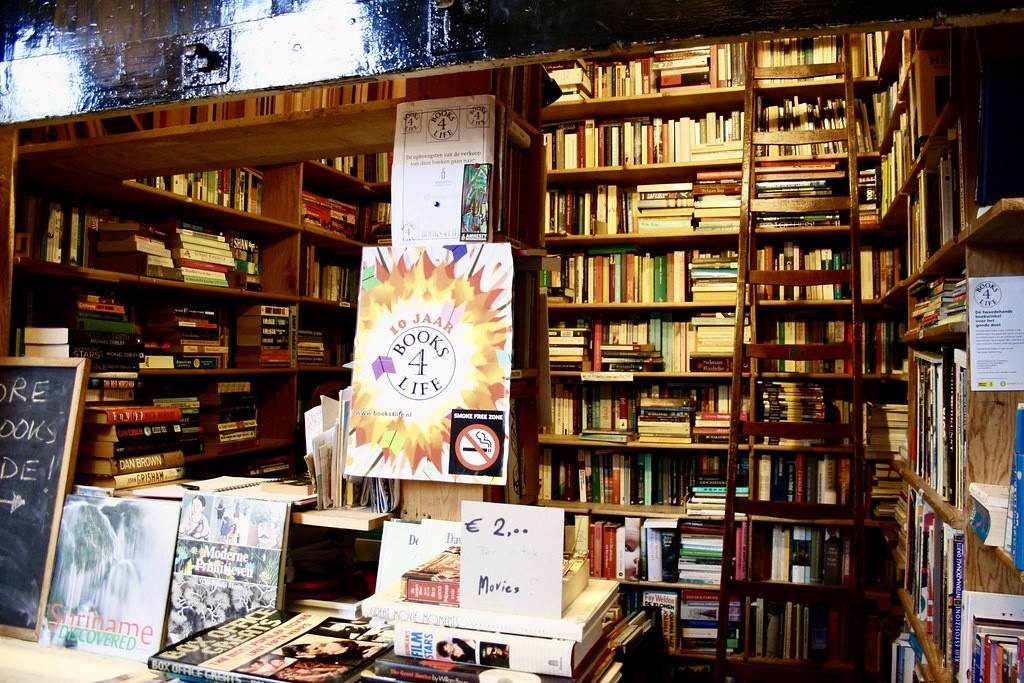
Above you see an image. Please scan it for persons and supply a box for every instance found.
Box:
[247,661,277,675]
[435,637,477,664]
[268,656,298,672]
[481,643,510,668]
[282,639,384,667]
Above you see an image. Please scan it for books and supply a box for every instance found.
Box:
[9,28,1024,682]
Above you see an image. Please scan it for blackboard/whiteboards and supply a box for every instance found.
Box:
[1,356,91,643]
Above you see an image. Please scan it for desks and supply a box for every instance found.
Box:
[0,636,180,683]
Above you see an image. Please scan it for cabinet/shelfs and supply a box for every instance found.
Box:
[506,7,1024,683]
[10,139,398,614]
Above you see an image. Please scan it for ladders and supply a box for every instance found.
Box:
[717,37,865,683]
